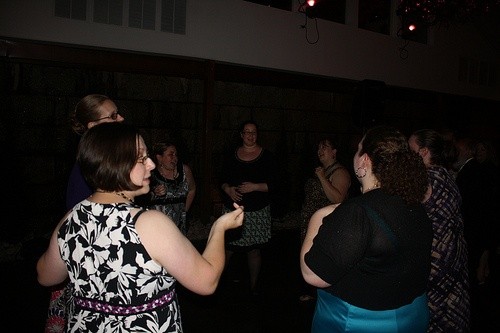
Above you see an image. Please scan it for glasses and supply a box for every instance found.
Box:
[92,110,123,122]
[241,131,257,135]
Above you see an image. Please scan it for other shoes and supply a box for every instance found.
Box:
[299,295,315,301]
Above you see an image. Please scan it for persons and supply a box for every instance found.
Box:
[477,137,496,163]
[44,94,167,333]
[298,125,433,333]
[299,135,352,303]
[214,120,283,304]
[408,133,472,333]
[444,129,500,333]
[35,120,243,333]
[149,137,197,234]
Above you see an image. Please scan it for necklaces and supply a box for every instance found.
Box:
[324,160,338,172]
[95,189,135,204]
[160,168,178,197]
